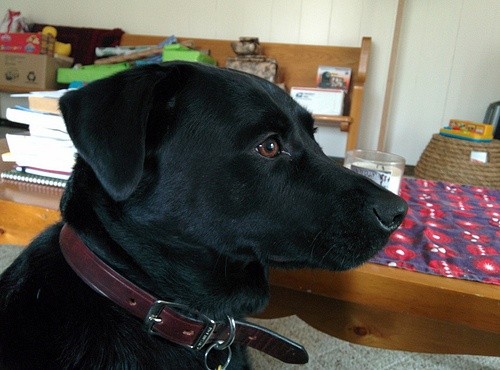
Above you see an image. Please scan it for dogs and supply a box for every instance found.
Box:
[0,60,407,370]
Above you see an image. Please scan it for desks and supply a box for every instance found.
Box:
[0,136,499,355]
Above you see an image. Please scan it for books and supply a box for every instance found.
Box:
[1,87,77,190]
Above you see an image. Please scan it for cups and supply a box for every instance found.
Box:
[343,147,406,197]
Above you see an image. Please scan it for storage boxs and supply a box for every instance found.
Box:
[0,53,64,93]
[0,32,54,56]
[289,87,344,116]
[314,65,352,92]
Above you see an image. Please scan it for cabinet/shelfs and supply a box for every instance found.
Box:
[33,24,372,155]
